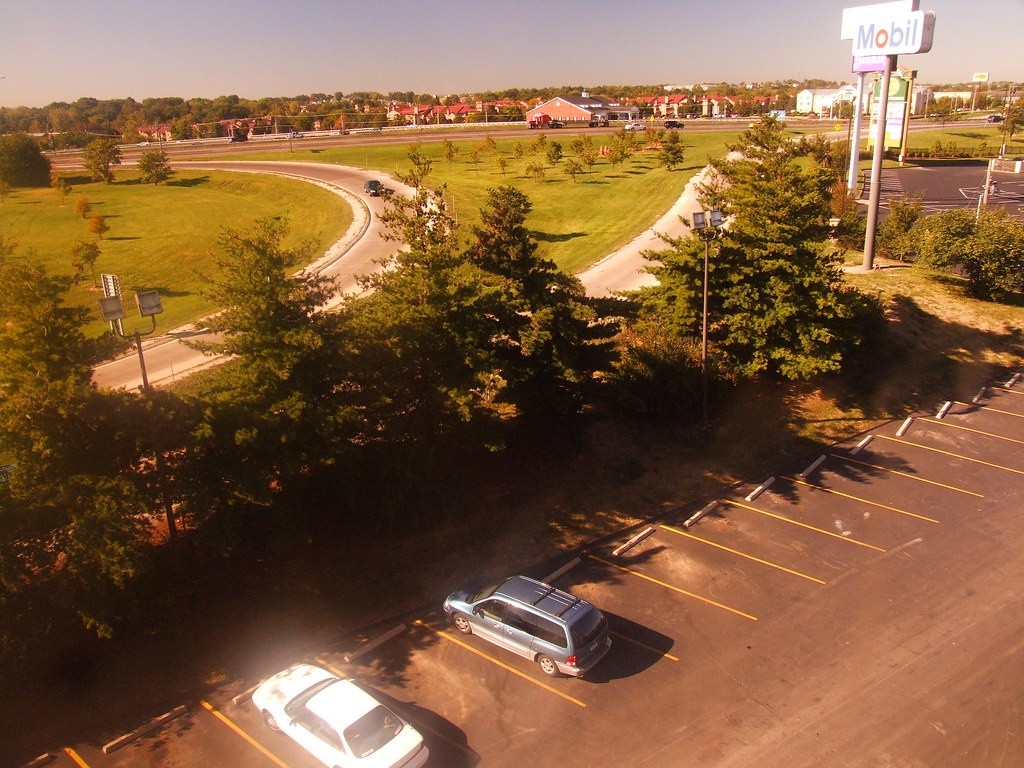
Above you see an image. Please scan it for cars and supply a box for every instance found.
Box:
[137,141,151,147]
[250,663,432,767]
[364,181,387,196]
[626,122,647,131]
[987,116,1004,124]
[288,133,304,138]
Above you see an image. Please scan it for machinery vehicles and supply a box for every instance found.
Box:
[529,114,563,129]
[588,113,611,127]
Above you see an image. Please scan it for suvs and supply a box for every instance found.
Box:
[443,574,614,678]
[664,120,685,130]
[228,134,249,143]
